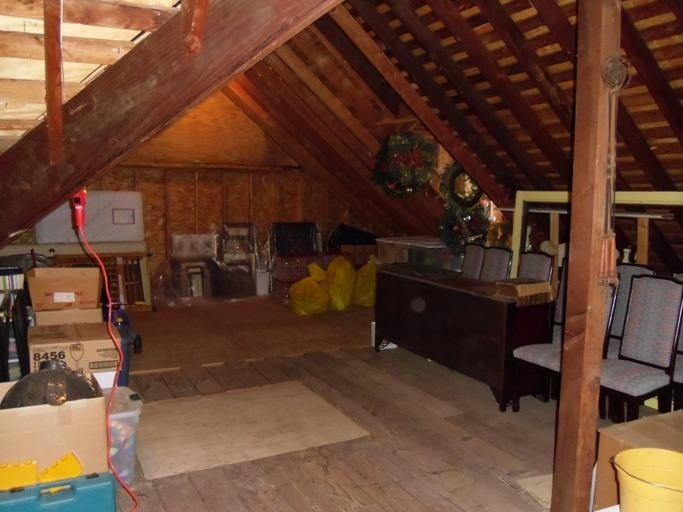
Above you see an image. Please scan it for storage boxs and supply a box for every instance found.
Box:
[26,266,103,311]
[26,322,121,392]
[594,409,683,512]
[0,372,109,492]
[34,305,103,326]
[372,231,457,270]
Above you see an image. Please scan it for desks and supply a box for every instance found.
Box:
[375,267,553,412]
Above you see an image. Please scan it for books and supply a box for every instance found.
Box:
[0,273,30,324]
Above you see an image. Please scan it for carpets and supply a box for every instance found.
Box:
[133,379,371,481]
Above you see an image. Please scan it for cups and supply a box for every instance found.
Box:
[609,447,683,512]
